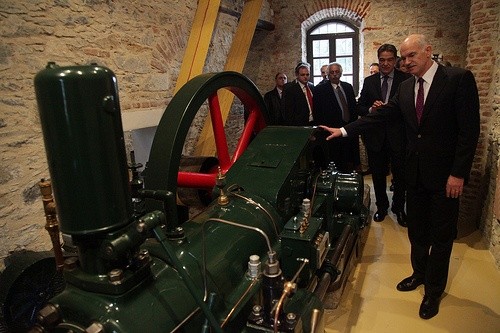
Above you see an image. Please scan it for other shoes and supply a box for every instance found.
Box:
[390,184,394,191]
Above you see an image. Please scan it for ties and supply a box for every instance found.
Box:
[305,85,313,112]
[415,77,424,123]
[336,86,350,122]
[381,75,390,103]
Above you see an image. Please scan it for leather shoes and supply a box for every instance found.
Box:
[396,275,425,292]
[373,209,388,222]
[391,206,408,227]
[419,291,442,319]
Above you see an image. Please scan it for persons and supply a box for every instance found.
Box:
[264,62,361,175]
[318,33,481,319]
[358,44,413,227]
[369,56,452,191]
